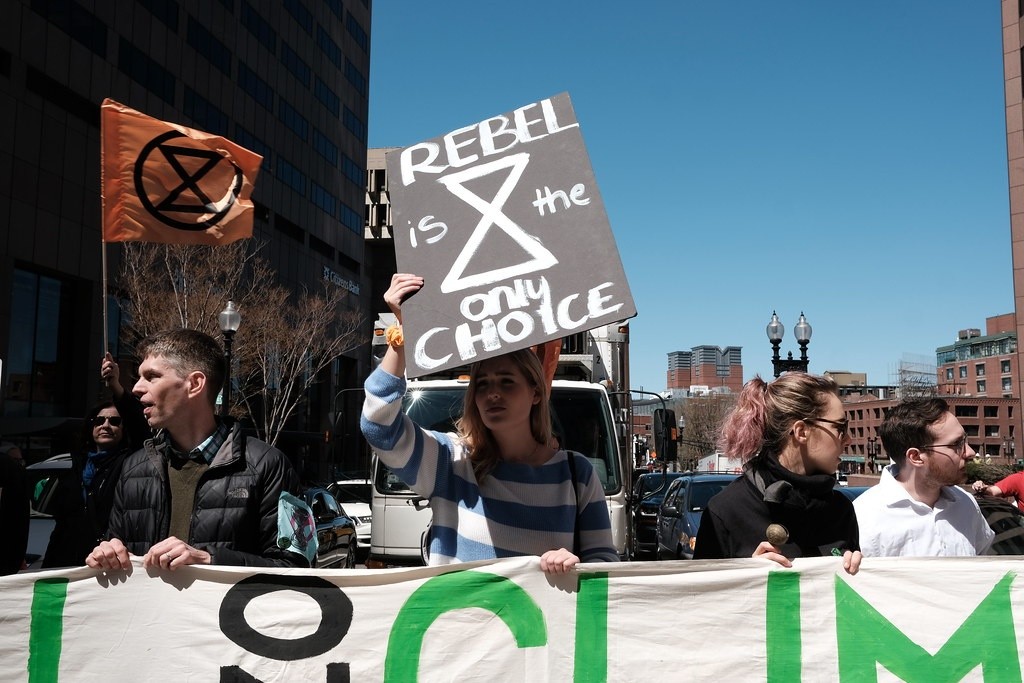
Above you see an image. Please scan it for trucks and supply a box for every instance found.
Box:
[322,311,679,568]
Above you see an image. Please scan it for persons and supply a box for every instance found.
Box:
[852,398,996,557]
[0,442,32,576]
[360,273,620,575]
[691,371,862,574]
[41,351,153,568]
[86,329,316,572]
[972,470,1024,511]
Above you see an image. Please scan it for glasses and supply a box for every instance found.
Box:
[90,417,123,425]
[916,433,967,450]
[790,417,848,435]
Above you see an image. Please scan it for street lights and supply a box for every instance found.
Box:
[865,434,879,473]
[219,300,242,417]
[1002,432,1015,464]
[678,415,685,447]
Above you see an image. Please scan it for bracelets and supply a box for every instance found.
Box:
[387,324,404,347]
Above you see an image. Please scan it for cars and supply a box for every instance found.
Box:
[295,488,357,569]
[631,466,694,555]
[24,453,73,570]
[655,471,743,560]
[324,479,373,548]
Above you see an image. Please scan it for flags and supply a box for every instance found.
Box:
[101,97,264,245]
